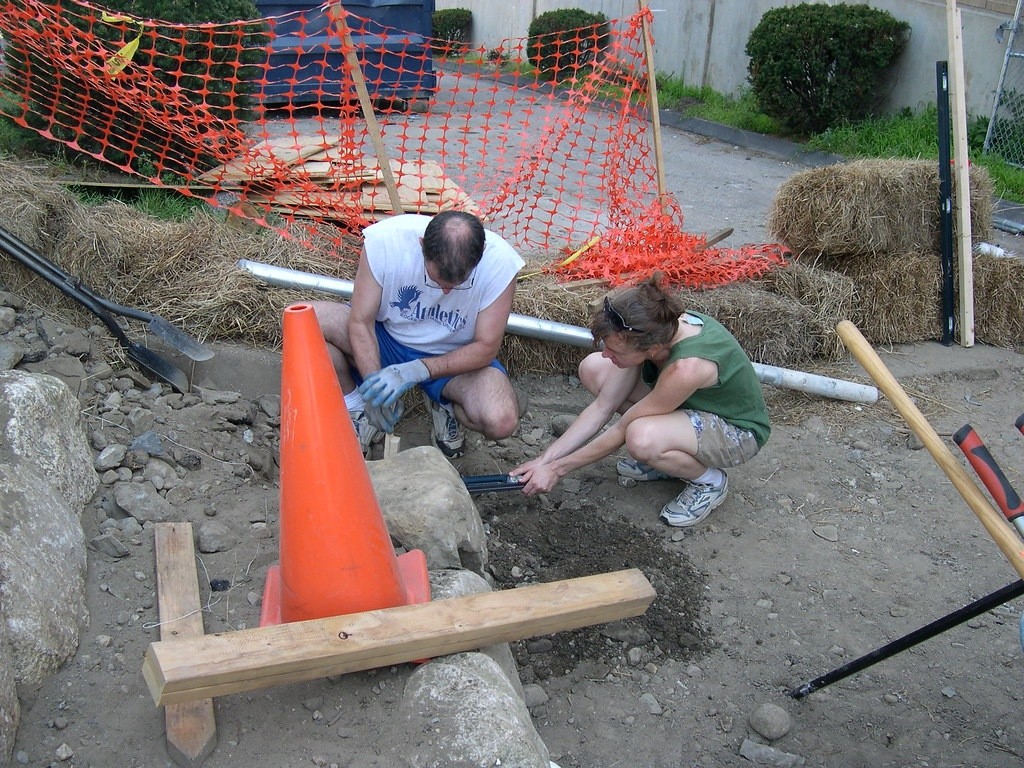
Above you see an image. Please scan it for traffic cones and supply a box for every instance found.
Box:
[251,302,429,662]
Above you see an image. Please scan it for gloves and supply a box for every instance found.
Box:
[358,358,431,409]
[363,396,405,434]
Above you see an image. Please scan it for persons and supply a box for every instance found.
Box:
[281,210,519,460]
[508,273,771,528]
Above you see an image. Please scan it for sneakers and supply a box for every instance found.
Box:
[422,391,467,459]
[659,468,728,528]
[616,460,669,482]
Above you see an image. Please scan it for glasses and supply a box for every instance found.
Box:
[603,296,646,334]
[423,263,477,290]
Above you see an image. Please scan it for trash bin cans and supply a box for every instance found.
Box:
[246,0,439,118]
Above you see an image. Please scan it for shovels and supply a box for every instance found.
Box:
[0,227,216,396]
